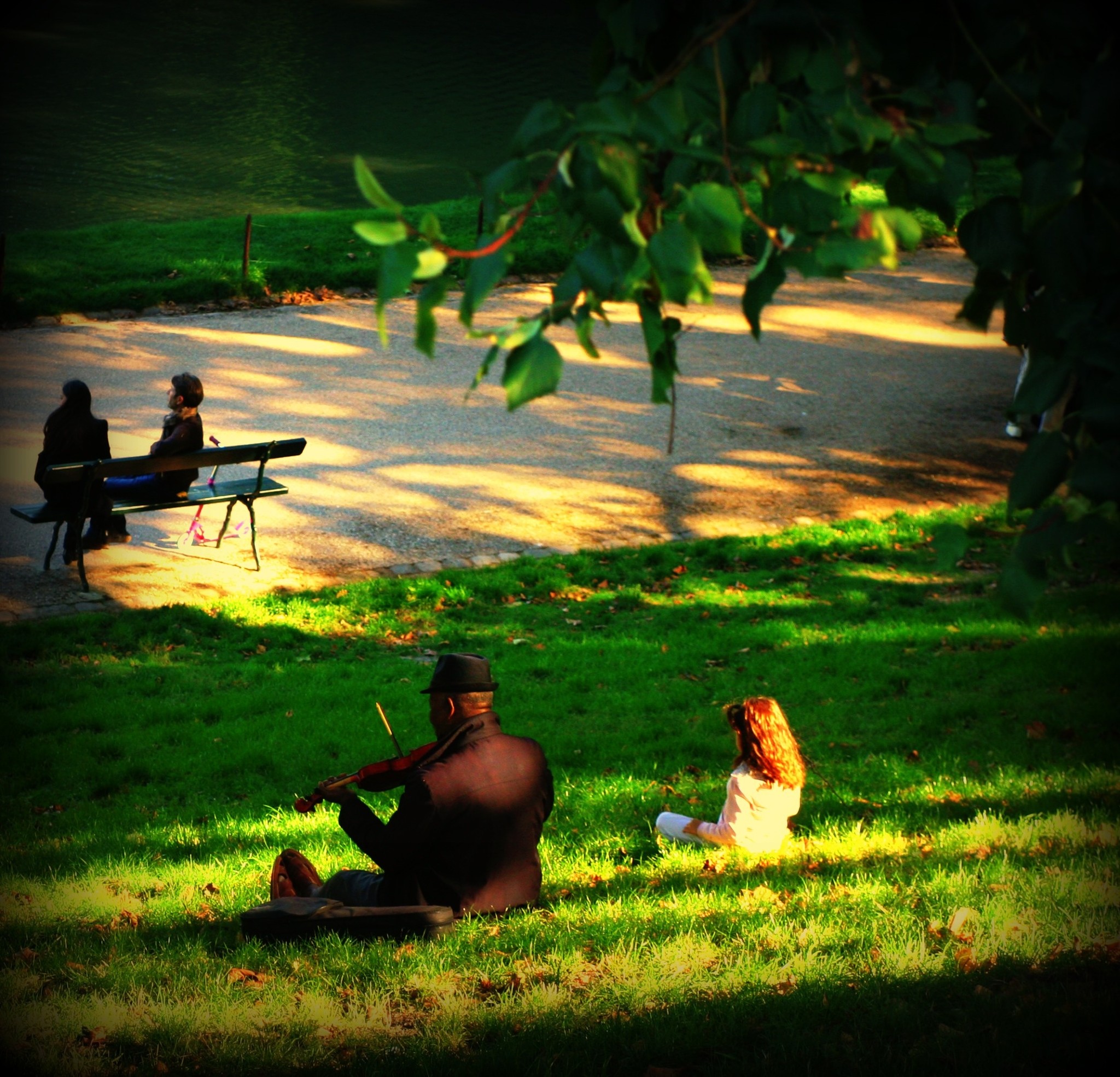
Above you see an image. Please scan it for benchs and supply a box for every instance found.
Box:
[9,438,307,592]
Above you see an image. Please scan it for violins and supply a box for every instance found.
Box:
[294,740,442,813]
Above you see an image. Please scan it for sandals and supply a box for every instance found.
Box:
[270,848,322,899]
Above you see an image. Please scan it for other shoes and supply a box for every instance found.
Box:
[80,535,107,550]
[63,545,79,566]
[105,534,132,543]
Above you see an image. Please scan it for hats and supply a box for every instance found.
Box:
[420,653,499,694]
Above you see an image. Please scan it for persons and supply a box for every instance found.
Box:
[654,698,804,850]
[269,654,555,920]
[44,378,111,566]
[83,373,204,548]
